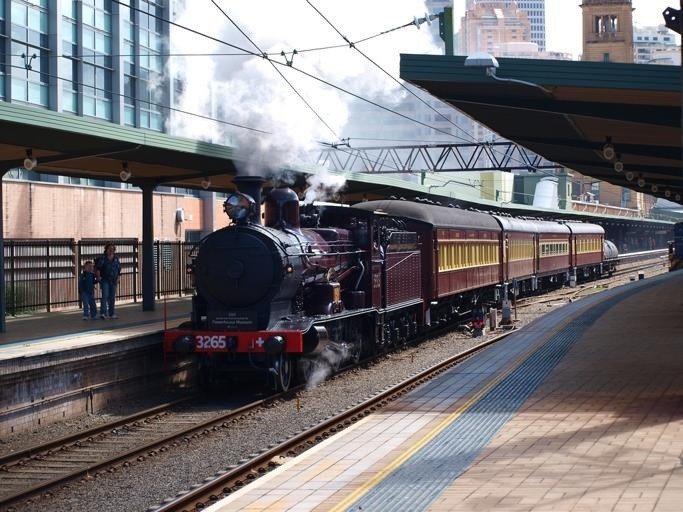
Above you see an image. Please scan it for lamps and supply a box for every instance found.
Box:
[464,50,498,77]
[117,167,131,184]
[592,138,681,205]
[22,157,37,172]
[200,181,212,191]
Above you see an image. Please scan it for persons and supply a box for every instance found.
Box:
[77,260,100,322]
[94,242,121,322]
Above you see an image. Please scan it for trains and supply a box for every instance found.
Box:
[666,221,682,272]
[161,177,619,396]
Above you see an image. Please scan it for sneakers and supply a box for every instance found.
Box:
[100,314,108,320]
[91,315,97,320]
[82,315,89,320]
[110,313,119,319]
